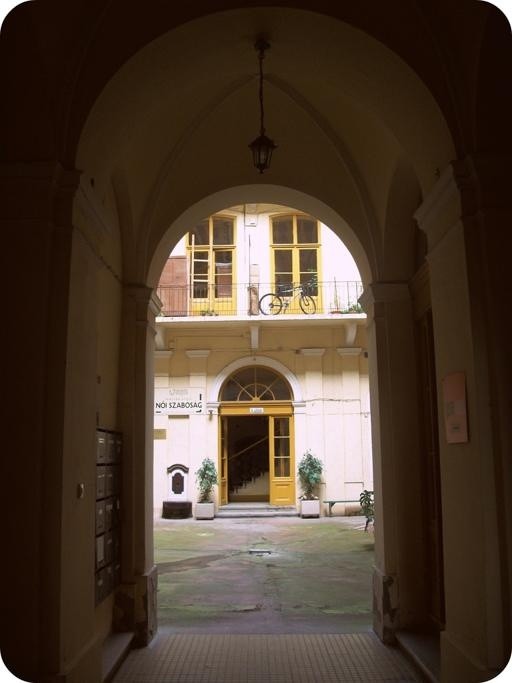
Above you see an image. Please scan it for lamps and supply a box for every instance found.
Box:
[247,41,279,175]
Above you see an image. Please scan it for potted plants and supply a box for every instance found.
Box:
[298,453,324,518]
[193,457,217,520]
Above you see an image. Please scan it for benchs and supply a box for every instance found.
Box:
[323,500,373,517]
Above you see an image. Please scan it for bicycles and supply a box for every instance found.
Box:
[259,281,317,315]
